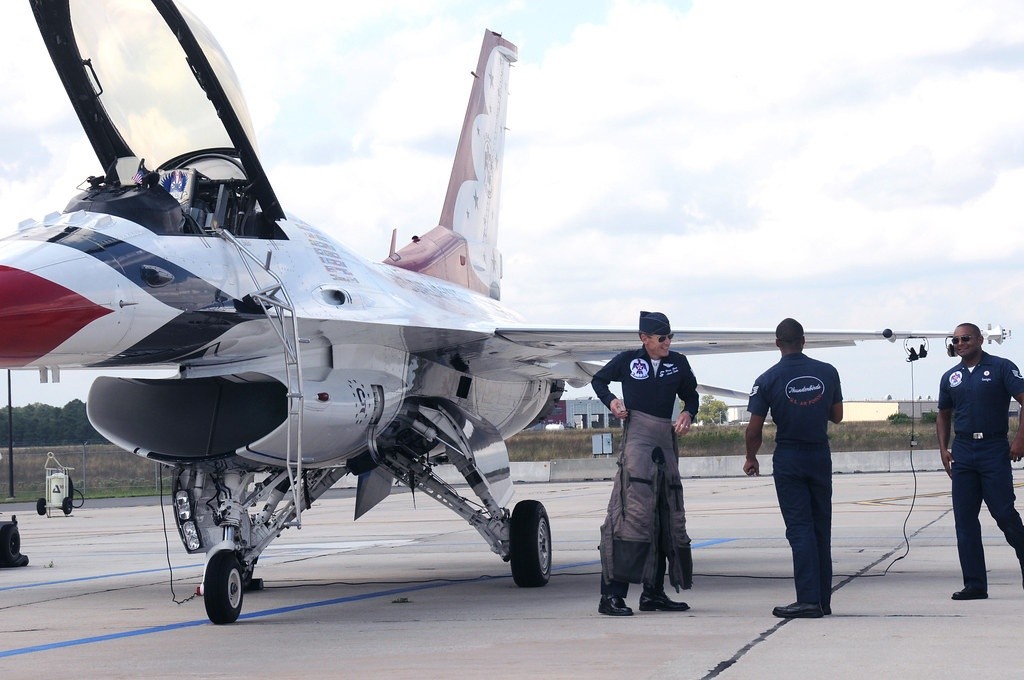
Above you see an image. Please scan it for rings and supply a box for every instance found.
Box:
[685,426,689,428]
[681,423,685,428]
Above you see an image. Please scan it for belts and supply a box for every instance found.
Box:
[955,432,1008,440]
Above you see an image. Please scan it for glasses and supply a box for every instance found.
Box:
[654,334,675,342]
[952,336,978,344]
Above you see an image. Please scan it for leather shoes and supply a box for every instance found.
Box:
[772,602,832,618]
[639,593,691,611]
[598,594,634,616]
[951,584,988,600]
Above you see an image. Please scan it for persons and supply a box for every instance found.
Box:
[937,323,1024,600]
[591,310,699,615]
[744,318,843,618]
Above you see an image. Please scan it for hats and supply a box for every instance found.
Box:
[639,311,671,335]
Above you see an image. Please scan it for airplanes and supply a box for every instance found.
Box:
[0,0,960,628]
